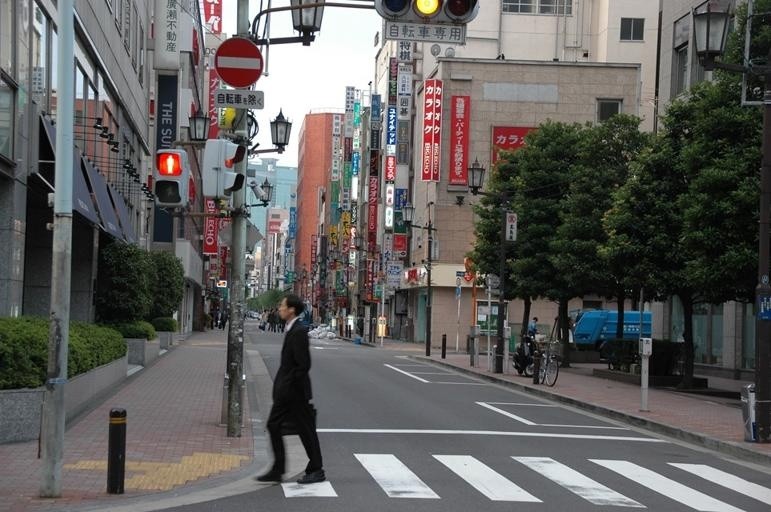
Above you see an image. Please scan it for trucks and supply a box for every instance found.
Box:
[573,308,653,360]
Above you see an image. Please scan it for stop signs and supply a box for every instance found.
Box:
[215,38,263,89]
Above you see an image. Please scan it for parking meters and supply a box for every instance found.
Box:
[638,338,654,414]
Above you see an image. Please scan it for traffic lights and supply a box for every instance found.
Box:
[153,149,193,210]
[376,1,481,25]
[201,136,246,202]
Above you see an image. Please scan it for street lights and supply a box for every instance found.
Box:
[292,261,318,324]
[226,0,329,438]
[246,176,275,207]
[465,157,511,372]
[402,200,440,355]
[247,108,295,156]
[352,231,375,343]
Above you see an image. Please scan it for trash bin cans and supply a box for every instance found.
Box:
[493,344,505,373]
[509,335,516,352]
[740,382,758,440]
[667,342,694,376]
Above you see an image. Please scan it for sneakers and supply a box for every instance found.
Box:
[297,470,325,484]
[256,471,283,483]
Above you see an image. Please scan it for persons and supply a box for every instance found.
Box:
[254,293,326,483]
[259,307,287,333]
[529,316,543,338]
[219,309,229,330]
[215,308,220,327]
[210,308,215,329]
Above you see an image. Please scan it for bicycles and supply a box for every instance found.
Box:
[539,339,562,387]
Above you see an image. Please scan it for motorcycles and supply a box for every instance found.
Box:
[513,331,538,378]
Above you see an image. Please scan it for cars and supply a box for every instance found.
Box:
[246,310,262,320]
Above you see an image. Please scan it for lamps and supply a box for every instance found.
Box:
[74,114,155,207]
